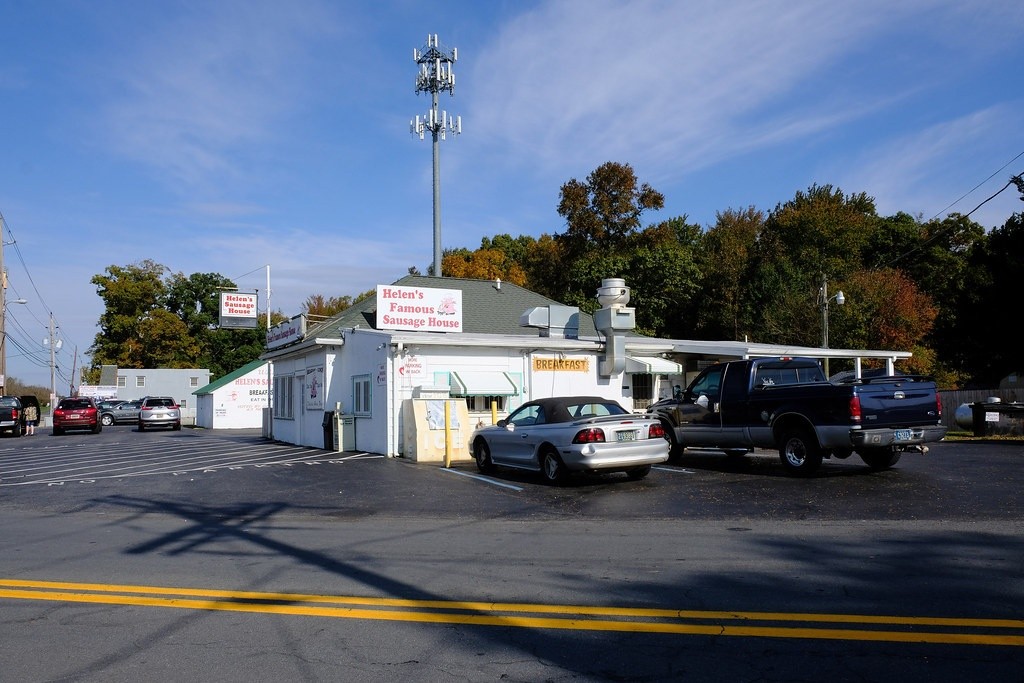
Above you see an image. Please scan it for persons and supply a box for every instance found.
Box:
[24,400,37,437]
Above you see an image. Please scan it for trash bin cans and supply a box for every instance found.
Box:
[968,401,1024,437]
[193,417,198,425]
[262,408,273,437]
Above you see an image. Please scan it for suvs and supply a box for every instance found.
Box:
[0,394,42,437]
[52,396,104,434]
[135,396,182,431]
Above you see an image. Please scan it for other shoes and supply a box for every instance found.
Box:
[30,432,33,436]
[24,433,28,436]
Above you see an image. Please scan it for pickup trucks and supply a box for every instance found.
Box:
[641,356,948,476]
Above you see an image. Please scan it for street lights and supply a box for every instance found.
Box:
[0,299,27,397]
[408,35,461,276]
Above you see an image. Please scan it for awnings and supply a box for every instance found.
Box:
[625,356,683,376]
[449,371,520,397]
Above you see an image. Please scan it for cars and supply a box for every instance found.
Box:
[102,401,140,426]
[468,396,670,483]
[95,399,129,409]
